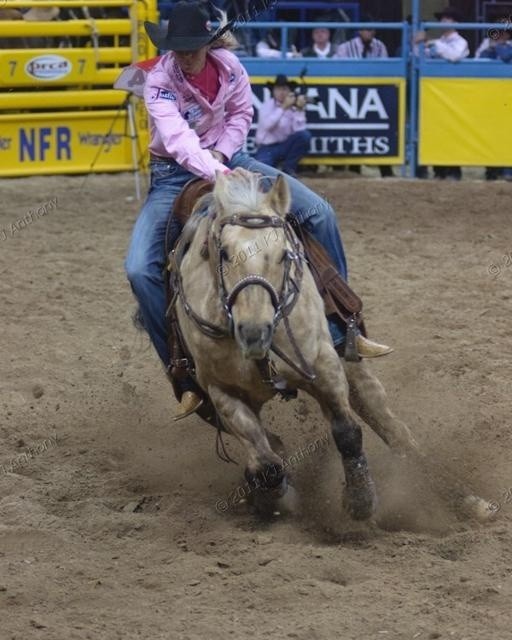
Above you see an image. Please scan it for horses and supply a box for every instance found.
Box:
[131,166,500,524]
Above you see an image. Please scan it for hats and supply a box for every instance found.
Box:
[144,2,236,52]
[434,8,464,23]
[266,75,298,97]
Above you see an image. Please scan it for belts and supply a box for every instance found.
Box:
[150,153,172,161]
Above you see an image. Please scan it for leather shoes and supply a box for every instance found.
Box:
[171,388,204,422]
[335,334,395,357]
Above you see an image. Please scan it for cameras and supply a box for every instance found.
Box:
[295,85,321,105]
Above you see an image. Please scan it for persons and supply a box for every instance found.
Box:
[123,1,394,421]
[241,9,511,184]
